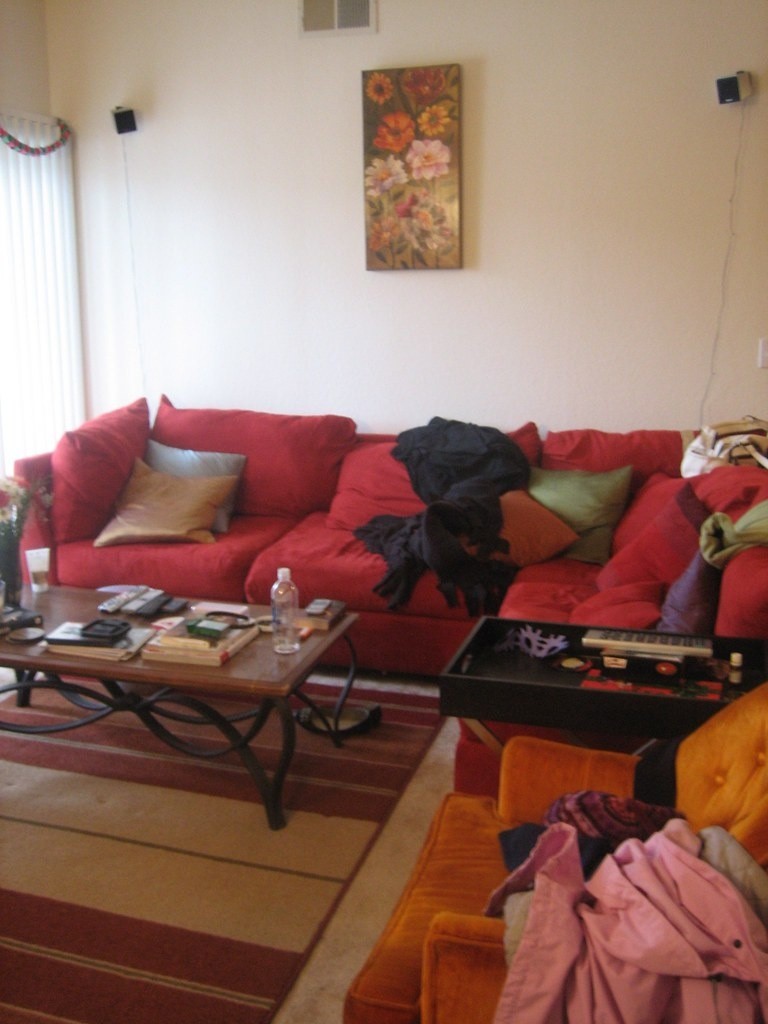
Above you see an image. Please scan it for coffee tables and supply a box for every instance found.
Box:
[0,583,360,832]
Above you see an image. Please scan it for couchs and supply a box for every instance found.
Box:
[451,464,768,798]
[334,684,768,1024]
[0,433,768,687]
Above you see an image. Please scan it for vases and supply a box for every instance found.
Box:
[0,534,23,608]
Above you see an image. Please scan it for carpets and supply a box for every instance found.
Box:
[0,674,447,1024]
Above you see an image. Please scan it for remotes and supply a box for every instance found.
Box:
[99,585,189,619]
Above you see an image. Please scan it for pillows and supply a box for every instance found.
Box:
[152,395,359,519]
[568,575,663,628]
[50,397,150,545]
[146,437,248,533]
[459,486,579,566]
[610,469,684,558]
[595,481,713,585]
[324,422,540,531]
[92,456,241,547]
[656,552,721,634]
[527,462,634,569]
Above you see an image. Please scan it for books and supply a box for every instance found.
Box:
[0,604,44,641]
[36,621,158,662]
[141,609,260,668]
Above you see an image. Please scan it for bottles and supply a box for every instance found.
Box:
[728,652,743,686]
[270,567,300,655]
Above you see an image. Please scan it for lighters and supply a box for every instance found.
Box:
[298,624,314,643]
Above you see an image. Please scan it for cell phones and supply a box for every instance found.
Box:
[306,599,331,615]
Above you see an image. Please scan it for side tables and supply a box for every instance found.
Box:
[438,613,768,809]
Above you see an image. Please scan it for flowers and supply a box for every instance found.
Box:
[0,470,55,539]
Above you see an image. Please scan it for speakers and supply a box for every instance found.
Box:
[112,108,137,135]
[716,72,752,104]
